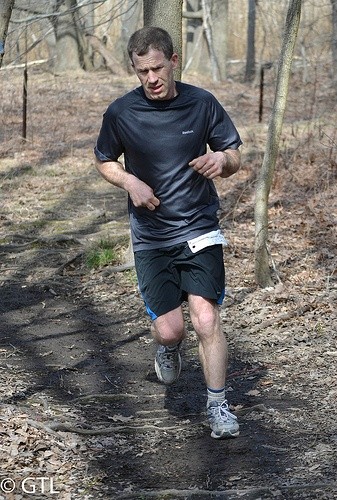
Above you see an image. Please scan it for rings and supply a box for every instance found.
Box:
[205,170,210,176]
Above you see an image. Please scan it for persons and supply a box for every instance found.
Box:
[93,27,244,439]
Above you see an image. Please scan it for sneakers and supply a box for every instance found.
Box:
[154,339,182,385]
[206,399,240,439]
